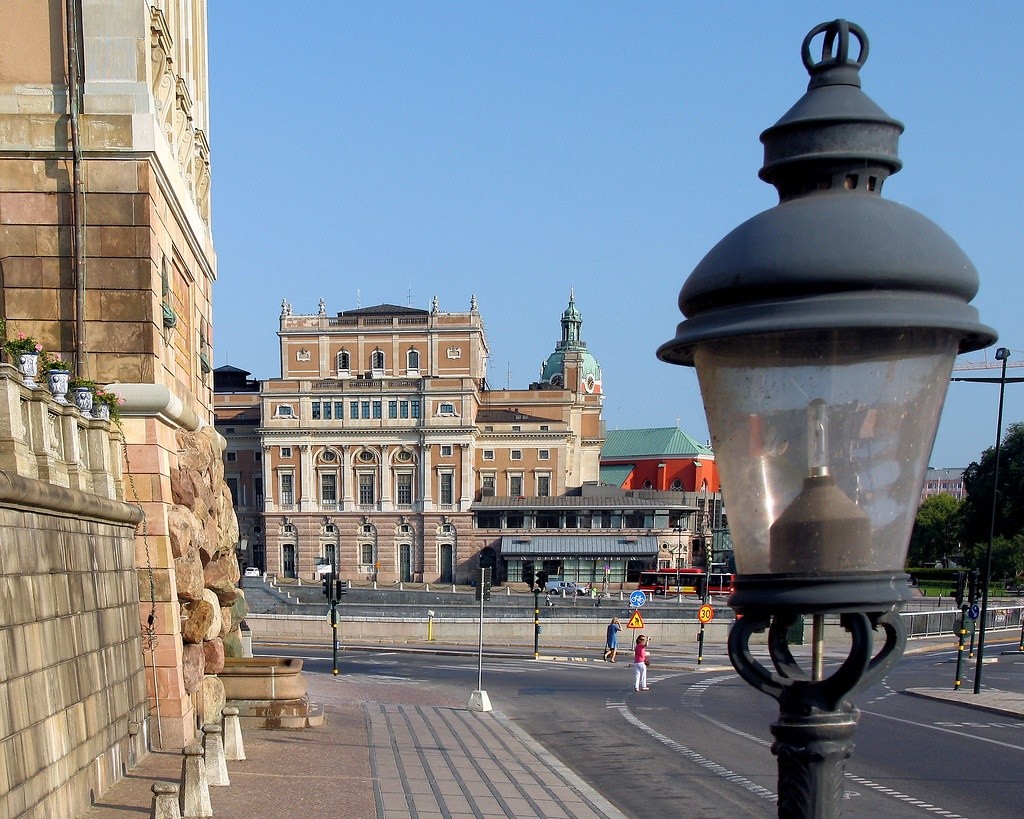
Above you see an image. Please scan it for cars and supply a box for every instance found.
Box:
[245,567,259,577]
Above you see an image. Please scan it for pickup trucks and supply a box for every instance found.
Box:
[544,580,586,596]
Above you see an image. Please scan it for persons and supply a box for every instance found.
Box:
[593,594,601,608]
[634,635,651,691]
[546,593,550,606]
[603,616,622,663]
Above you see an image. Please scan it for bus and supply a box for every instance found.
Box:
[638,568,737,596]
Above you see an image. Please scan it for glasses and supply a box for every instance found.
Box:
[640,639,646,641]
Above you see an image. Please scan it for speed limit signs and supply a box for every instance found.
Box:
[698,605,713,623]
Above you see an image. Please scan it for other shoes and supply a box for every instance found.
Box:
[610,660,616,663]
[635,688,640,692]
[642,687,649,691]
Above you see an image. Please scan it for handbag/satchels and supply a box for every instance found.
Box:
[603,643,612,659]
[644,659,650,666]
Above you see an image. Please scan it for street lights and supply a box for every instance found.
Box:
[673,526,687,568]
[974,346,1011,693]
[657,19,1000,819]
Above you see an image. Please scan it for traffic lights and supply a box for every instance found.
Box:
[322,575,347,605]
[525,564,546,596]
[950,567,982,611]
